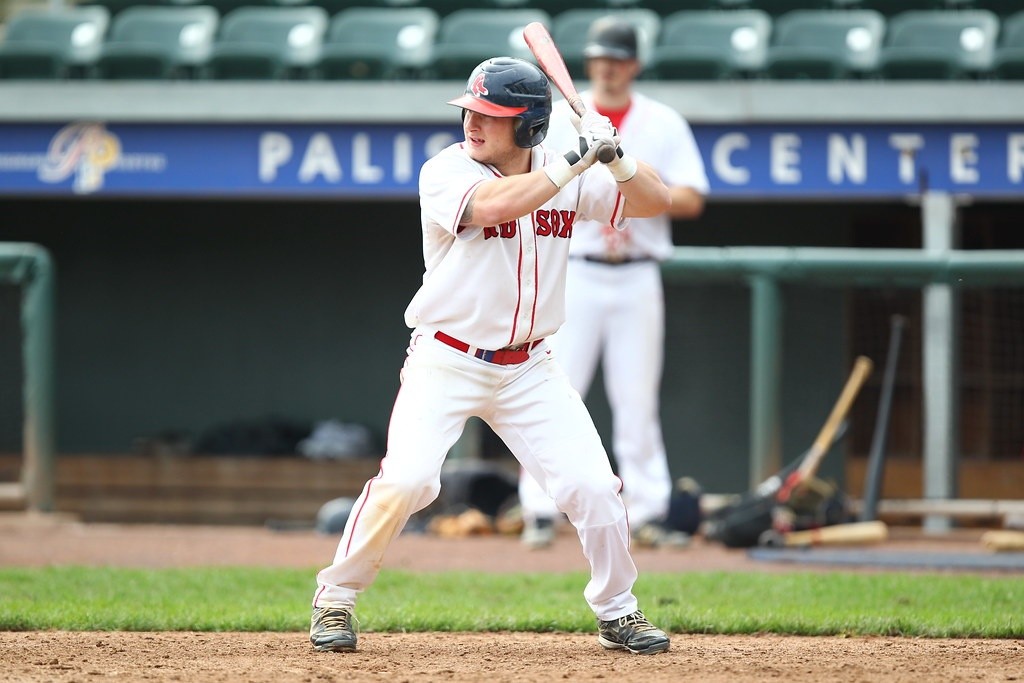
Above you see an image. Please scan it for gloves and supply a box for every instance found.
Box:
[570,112,637,181]
[543,113,621,188]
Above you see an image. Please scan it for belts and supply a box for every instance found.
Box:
[434,331,544,364]
[570,256,652,264]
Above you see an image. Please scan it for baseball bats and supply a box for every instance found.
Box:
[981,529,1024,554]
[700,420,850,522]
[758,519,890,550]
[774,356,875,503]
[523,21,616,164]
[858,313,910,521]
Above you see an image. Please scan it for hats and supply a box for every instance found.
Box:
[584,16,638,60]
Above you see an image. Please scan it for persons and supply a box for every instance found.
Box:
[309,16,711,655]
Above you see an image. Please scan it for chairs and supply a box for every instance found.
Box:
[0,4,1024,82]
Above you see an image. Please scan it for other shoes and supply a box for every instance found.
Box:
[631,524,662,548]
[521,517,553,550]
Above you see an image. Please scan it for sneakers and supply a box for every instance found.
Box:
[310,607,357,650]
[597,610,670,654]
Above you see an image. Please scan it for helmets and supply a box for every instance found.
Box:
[446,56,552,148]
